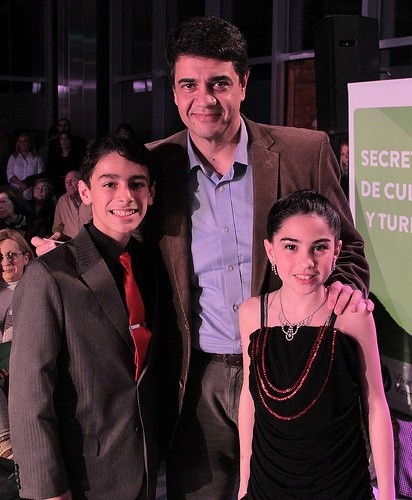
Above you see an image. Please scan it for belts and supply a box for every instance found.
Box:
[196,350,243,368]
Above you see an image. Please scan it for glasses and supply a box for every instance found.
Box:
[0,250,25,262]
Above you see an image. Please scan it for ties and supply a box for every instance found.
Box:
[118,252,152,381]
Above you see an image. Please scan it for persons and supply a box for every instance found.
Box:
[9,134,183,500]
[237,189,395,500]
[337,142,349,199]
[0,229,34,459]
[0,121,134,245]
[31,18,374,500]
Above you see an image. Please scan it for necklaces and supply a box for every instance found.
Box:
[276,286,327,340]
